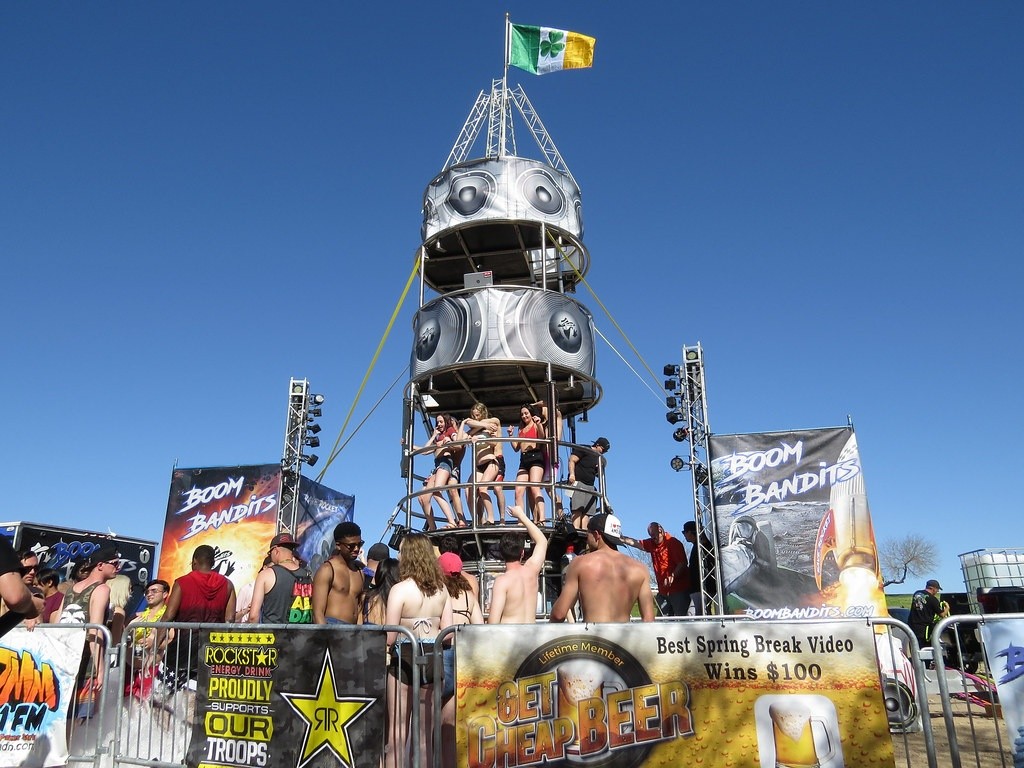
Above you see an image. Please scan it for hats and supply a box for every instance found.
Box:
[81,546,120,571]
[291,549,308,568]
[437,551,464,578]
[586,512,628,549]
[926,580,943,590]
[367,542,389,561]
[269,533,300,549]
[591,437,610,450]
[257,555,273,573]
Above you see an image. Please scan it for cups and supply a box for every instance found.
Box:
[769,707,836,768]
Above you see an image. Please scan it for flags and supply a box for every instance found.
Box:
[508,22,595,76]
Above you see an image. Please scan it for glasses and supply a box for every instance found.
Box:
[143,589,165,596]
[267,547,278,556]
[599,446,608,454]
[102,561,120,568]
[521,402,532,408]
[336,539,365,549]
[24,565,39,574]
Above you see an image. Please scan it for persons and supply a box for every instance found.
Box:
[909,580,949,670]
[670,520,717,621]
[620,521,691,622]
[486,505,548,624]
[550,514,655,624]
[0,532,236,736]
[310,521,483,768]
[399,402,563,531]
[236,533,314,624]
[568,437,610,529]
